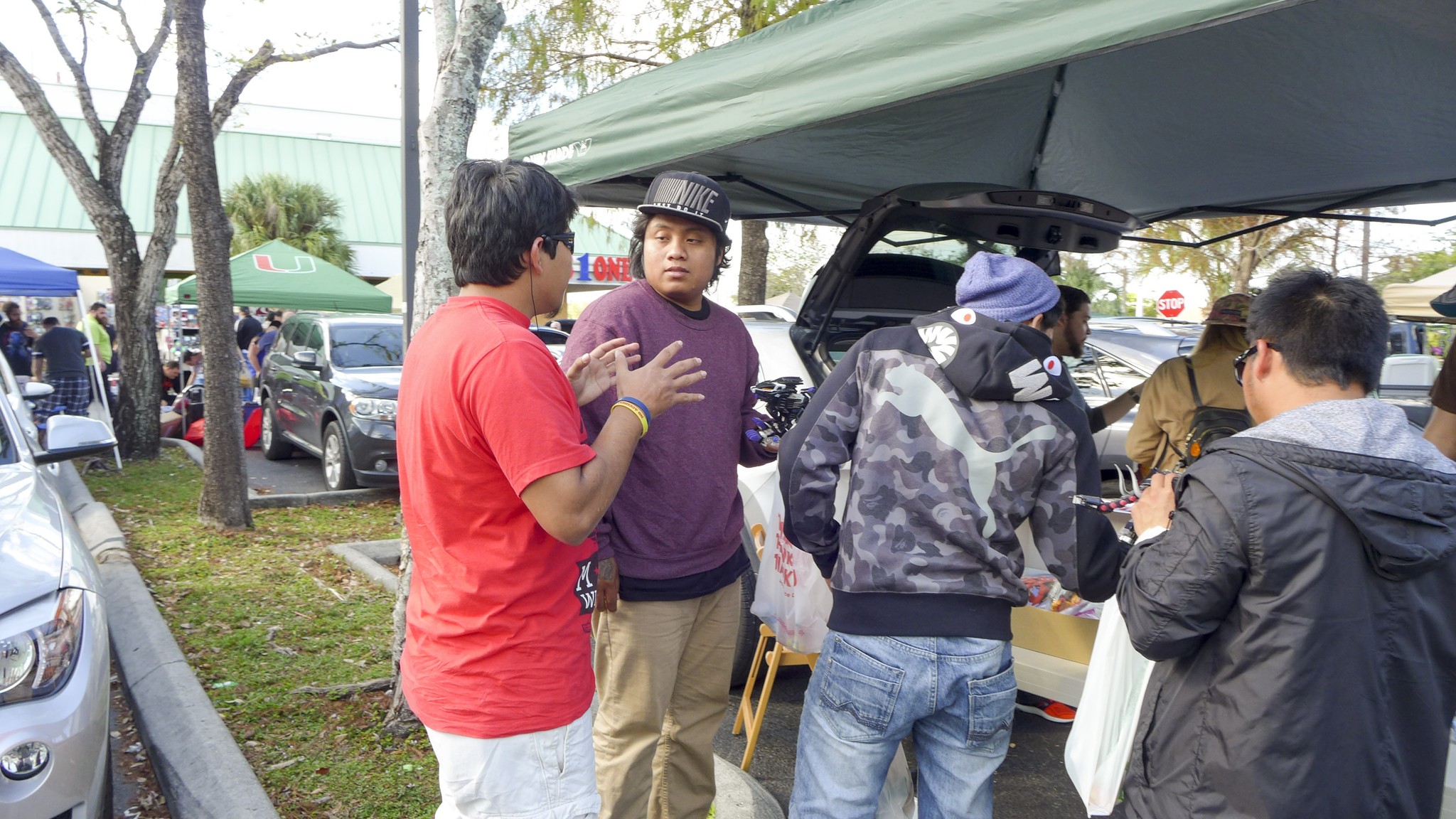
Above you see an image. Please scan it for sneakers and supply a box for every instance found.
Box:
[1015,689,1077,722]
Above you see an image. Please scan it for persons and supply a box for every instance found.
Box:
[396,155,706,819]
[0,296,296,444]
[778,250,1134,819]
[1124,294,1260,482]
[1117,265,1456,819]
[1421,285,1456,463]
[1050,285,1147,434]
[561,170,783,819]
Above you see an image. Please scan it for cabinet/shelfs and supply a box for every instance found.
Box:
[168,304,239,437]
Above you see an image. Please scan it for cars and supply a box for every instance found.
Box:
[1,353,119,819]
[525,177,1447,482]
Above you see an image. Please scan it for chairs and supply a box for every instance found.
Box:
[732,524,821,772]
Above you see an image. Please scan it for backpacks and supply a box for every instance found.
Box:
[1176,356,1251,469]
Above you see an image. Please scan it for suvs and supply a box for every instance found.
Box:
[260,310,400,492]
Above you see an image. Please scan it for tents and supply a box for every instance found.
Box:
[502,0,1456,249]
[163,238,392,436]
[0,246,122,468]
[1381,266,1456,326]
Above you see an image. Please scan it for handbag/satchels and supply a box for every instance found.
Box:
[751,449,834,655]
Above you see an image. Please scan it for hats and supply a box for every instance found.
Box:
[637,170,731,236]
[955,252,1061,323]
[1431,285,1456,318]
[1202,293,1255,328]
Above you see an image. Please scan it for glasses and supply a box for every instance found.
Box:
[1232,342,1282,387]
[529,231,575,254]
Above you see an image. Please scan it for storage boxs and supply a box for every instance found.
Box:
[158,411,183,438]
[1010,568,1099,665]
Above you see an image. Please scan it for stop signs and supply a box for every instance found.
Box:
[1157,289,1185,318]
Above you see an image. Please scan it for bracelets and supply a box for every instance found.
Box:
[611,401,648,439]
[1128,388,1139,404]
[613,396,651,427]
